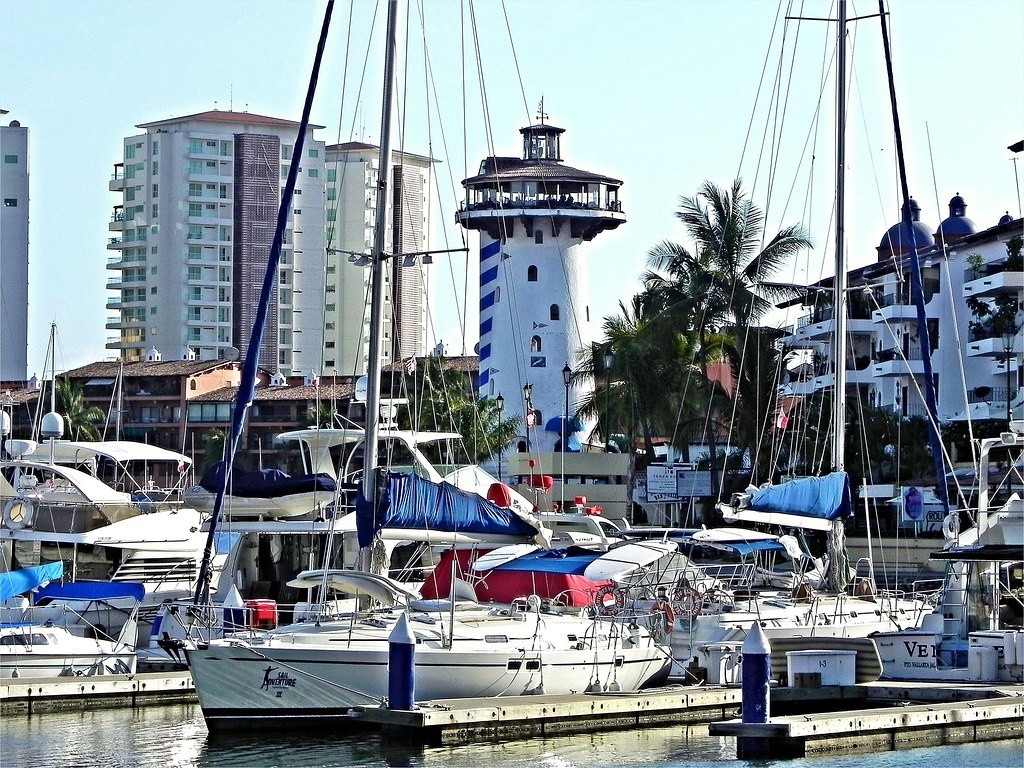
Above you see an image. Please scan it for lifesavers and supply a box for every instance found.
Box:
[672,587,702,618]
[594,584,625,616]
[650,602,674,635]
[3,496,33,530]
[942,514,960,539]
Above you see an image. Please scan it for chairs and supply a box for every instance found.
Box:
[916,613,948,667]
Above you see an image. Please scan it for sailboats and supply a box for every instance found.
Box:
[1,0,1024,733]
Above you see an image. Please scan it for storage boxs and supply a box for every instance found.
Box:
[245,600,277,630]
[937,640,969,667]
